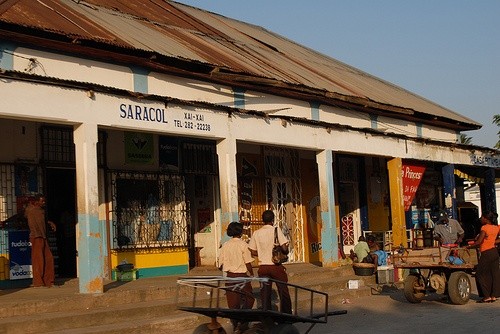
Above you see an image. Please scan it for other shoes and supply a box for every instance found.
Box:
[30,284,56,289]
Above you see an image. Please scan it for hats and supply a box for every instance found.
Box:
[437,210,449,222]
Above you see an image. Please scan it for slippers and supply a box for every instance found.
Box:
[476,297,497,303]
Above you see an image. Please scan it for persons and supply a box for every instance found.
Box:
[431,212,464,299]
[467,209,500,302]
[217,221,254,334]
[24,193,56,289]
[351,235,380,273]
[248,210,292,316]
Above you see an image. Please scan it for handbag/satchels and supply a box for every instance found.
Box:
[272,227,289,264]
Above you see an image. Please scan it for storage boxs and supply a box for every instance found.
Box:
[349,280,360,290]
[112,269,136,281]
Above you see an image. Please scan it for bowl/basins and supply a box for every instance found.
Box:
[354,263,375,276]
